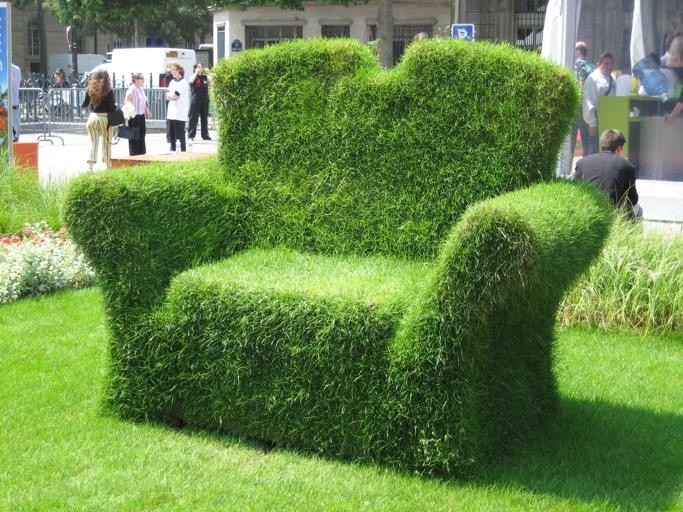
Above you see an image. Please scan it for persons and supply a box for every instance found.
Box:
[569,40,589,160]
[123,72,152,157]
[639,27,683,127]
[187,61,213,143]
[50,67,70,88]
[571,124,647,236]
[12,63,20,143]
[161,64,188,152]
[580,49,616,155]
[159,62,178,87]
[76,65,115,171]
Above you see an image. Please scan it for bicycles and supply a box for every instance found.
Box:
[21,69,85,119]
[106,105,121,145]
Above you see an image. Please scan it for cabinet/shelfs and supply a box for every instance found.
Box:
[598,94,681,162]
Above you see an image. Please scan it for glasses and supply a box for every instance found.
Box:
[134,77,144,80]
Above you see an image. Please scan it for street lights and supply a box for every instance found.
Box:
[66,18,82,89]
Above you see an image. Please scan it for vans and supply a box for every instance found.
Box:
[73,46,197,109]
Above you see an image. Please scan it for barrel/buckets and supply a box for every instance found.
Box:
[615,74,631,96]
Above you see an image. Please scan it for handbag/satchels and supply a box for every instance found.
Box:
[107,106,141,145]
